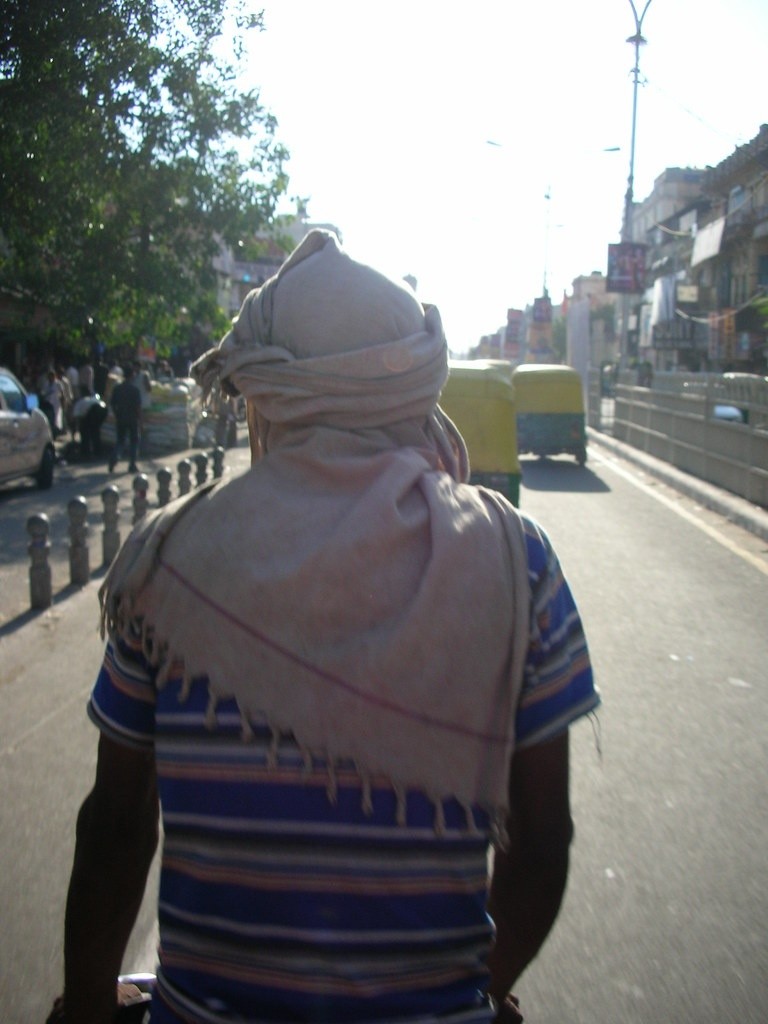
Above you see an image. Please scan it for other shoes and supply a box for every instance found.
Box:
[108,458,117,472]
[128,464,139,473]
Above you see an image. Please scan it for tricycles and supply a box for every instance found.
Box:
[438,358,523,509]
[512,362,589,466]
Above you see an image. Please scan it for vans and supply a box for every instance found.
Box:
[1,365,57,490]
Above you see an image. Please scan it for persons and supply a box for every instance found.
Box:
[42,227,603,1023]
[638,352,653,387]
[37,352,153,473]
